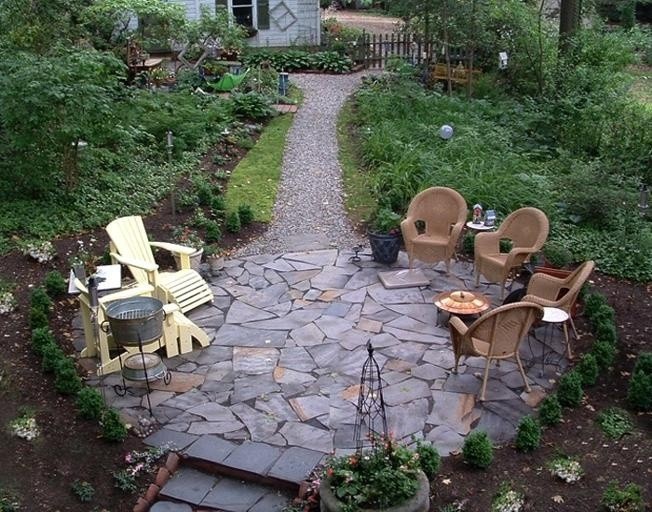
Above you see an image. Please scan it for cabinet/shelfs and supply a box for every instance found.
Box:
[99,309,172,417]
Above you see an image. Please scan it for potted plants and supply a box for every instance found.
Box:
[365,207,404,263]
[164,223,205,270]
[203,243,226,271]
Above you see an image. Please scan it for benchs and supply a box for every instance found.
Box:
[125,33,164,89]
[432,62,470,84]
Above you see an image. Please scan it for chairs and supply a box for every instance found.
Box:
[400,186,467,277]
[472,206,549,302]
[105,213,214,316]
[72,276,212,376]
[449,301,545,402]
[516,258,597,359]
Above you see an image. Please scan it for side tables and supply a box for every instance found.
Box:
[526,307,568,377]
[466,221,495,230]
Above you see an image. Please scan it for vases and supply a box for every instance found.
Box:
[317,467,431,512]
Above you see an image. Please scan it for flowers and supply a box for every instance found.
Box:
[322,432,420,512]
